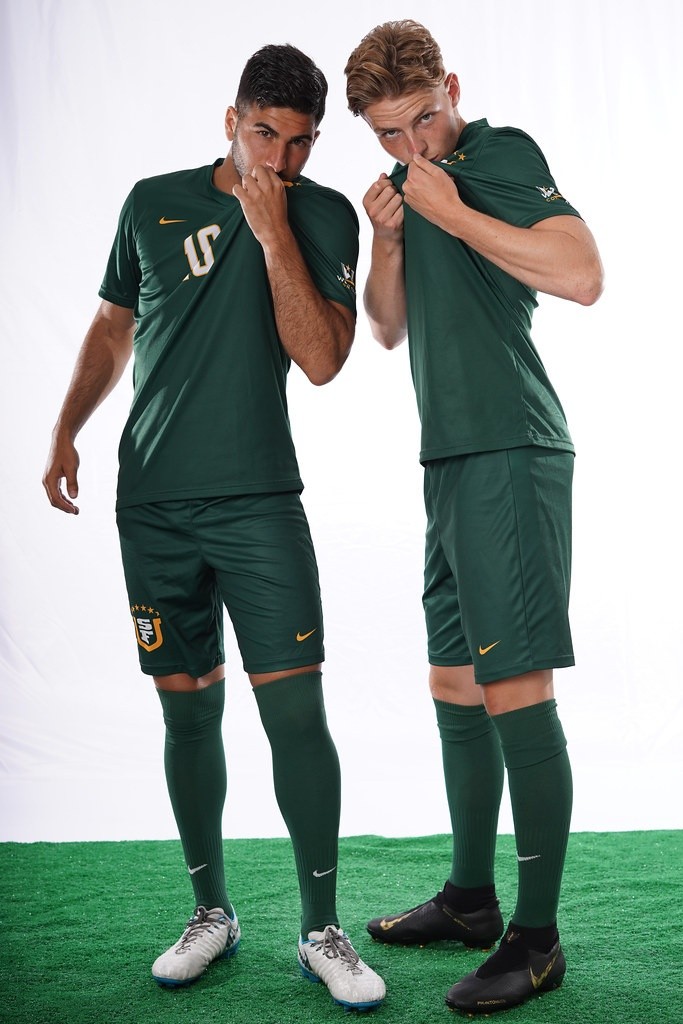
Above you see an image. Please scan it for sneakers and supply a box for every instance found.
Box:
[294,925,387,1013]
[442,936,566,1019]
[366,893,504,951]
[151,902,240,989]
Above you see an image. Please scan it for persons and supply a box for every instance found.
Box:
[343,20,603,1013]
[39,41,388,1009]
